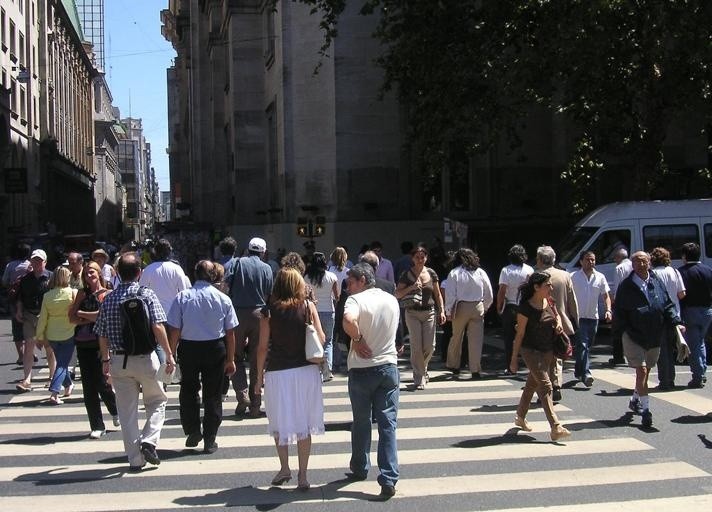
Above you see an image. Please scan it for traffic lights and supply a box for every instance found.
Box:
[298,216,326,237]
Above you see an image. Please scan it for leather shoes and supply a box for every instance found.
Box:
[553,386,562,401]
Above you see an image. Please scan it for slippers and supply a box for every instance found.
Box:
[271,473,310,488]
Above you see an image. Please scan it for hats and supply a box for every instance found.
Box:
[91,249,109,263]
[249,237,267,252]
[30,249,47,261]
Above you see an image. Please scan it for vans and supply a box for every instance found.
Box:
[530,198,712,324]
[63,233,95,265]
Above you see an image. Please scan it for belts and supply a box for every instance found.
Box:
[113,351,127,355]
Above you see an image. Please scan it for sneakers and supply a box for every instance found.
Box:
[17,355,38,391]
[382,485,395,495]
[551,427,570,440]
[45,378,74,404]
[497,369,516,378]
[609,359,703,425]
[113,415,120,426]
[130,443,160,468]
[575,373,594,387]
[236,399,260,415]
[417,368,480,389]
[515,416,531,431]
[186,430,218,453]
[91,430,106,439]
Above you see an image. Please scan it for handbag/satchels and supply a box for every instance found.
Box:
[548,298,572,360]
[305,299,324,362]
[399,268,422,308]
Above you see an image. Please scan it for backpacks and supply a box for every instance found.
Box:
[74,288,107,321]
[119,285,157,355]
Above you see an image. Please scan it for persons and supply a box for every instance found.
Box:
[0,236,712,495]
